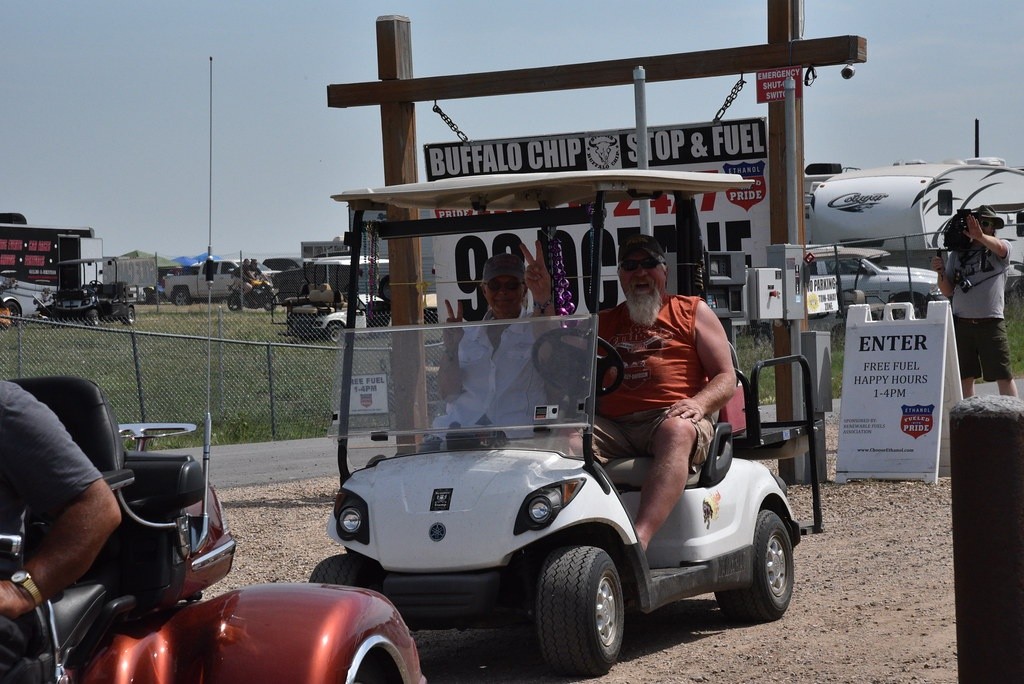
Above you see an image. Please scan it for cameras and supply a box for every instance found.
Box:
[958,278,972,293]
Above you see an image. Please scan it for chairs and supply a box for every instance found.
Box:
[602,308,743,488]
[0,375,136,684]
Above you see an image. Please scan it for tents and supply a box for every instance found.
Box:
[118,251,224,298]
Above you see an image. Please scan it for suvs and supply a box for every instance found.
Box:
[804,247,943,319]
[261,256,305,270]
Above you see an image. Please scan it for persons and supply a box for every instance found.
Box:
[932,205,1019,400]
[232,259,268,301]
[419,252,549,450]
[520,234,736,551]
[0,380,124,684]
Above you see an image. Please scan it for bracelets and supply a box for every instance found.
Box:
[533,296,552,317]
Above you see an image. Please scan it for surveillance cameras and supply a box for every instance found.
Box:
[841,65,855,80]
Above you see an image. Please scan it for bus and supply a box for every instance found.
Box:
[0,222,96,326]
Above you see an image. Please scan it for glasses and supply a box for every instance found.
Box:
[484,279,524,292]
[617,256,666,271]
[978,219,994,228]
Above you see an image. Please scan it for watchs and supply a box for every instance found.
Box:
[11,569,42,606]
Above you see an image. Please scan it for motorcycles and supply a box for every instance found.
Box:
[225,272,280,312]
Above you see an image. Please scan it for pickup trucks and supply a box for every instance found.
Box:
[162,258,277,307]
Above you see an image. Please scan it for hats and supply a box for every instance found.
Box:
[977,204,1004,230]
[616,235,666,270]
[483,253,526,284]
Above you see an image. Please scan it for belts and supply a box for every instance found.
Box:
[958,318,992,325]
